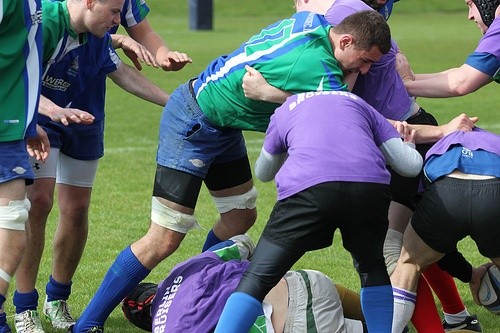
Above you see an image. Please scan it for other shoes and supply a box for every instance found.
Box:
[66,325,104,333]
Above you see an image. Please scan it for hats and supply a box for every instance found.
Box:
[474,1,500,27]
[122,282,157,332]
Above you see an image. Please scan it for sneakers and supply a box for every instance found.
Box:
[42,295,76,327]
[12,310,46,333]
[440,315,481,333]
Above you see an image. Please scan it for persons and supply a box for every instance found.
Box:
[121,234,410,333]
[296,0,482,333]
[108,0,192,72]
[73,10,480,333]
[394,0,500,98]
[0,0,50,333]
[13,33,170,333]
[388,129,500,333]
[212,90,424,333]
[26,0,127,164]
